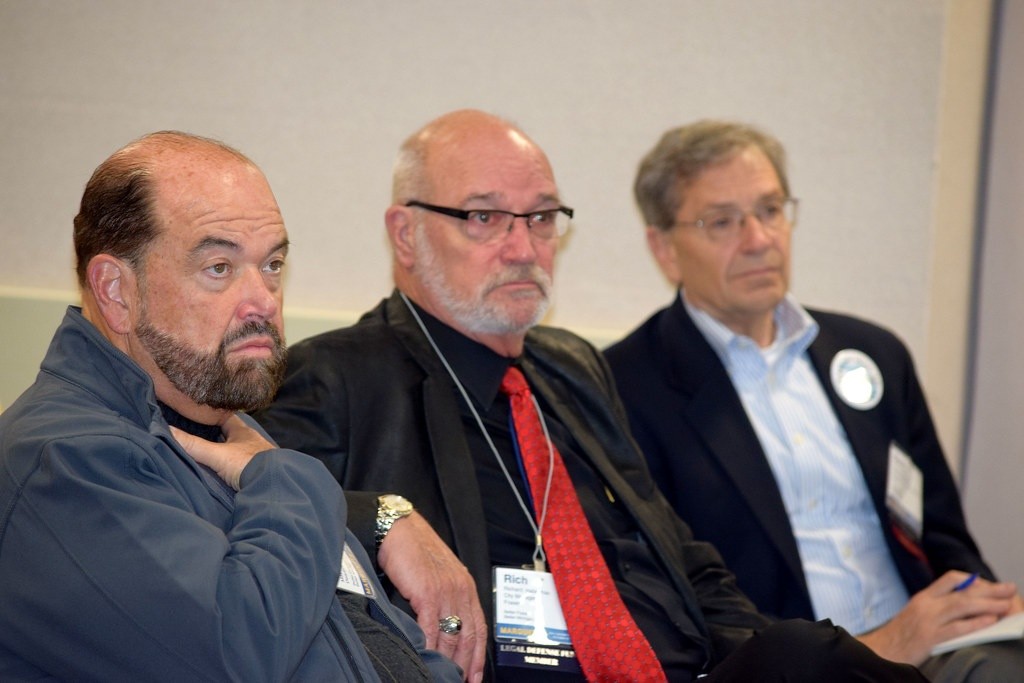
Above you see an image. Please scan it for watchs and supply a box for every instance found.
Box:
[372,494,414,558]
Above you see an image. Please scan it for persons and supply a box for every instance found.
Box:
[601,121,1024,683]
[3,129,485,683]
[249,110,933,683]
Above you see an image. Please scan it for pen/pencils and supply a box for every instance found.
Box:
[951,569,982,592]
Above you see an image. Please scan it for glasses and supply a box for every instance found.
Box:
[405,199,575,242]
[661,197,799,244]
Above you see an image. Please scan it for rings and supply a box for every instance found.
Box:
[439,615,463,635]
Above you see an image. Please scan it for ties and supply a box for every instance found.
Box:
[500,365,669,683]
